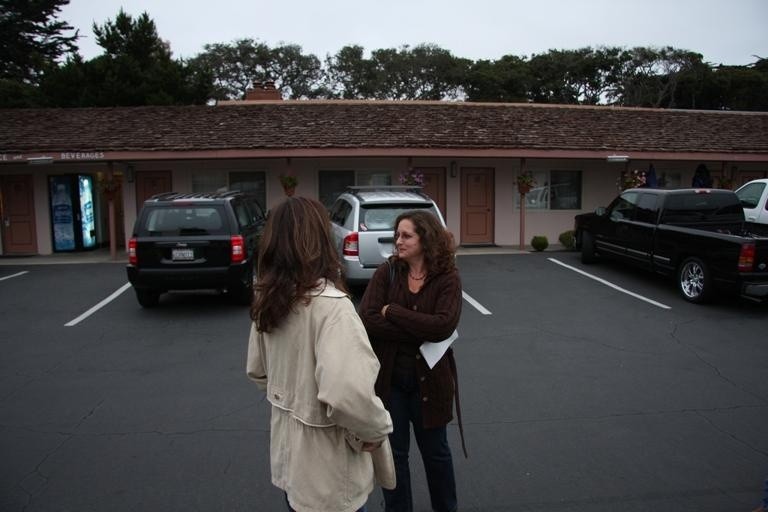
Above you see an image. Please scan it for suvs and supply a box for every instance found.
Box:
[125,190,268,308]
[328,186,457,293]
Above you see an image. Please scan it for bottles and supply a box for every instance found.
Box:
[52,184,75,251]
[81,179,95,248]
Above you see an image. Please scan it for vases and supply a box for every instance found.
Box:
[519,183,529,193]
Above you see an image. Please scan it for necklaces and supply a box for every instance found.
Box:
[407,270,428,282]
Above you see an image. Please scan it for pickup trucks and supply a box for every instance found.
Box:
[574,188,767,303]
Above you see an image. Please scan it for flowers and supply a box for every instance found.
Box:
[512,169,540,189]
[616,169,647,191]
[399,167,426,193]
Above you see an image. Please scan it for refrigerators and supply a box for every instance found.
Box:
[47,172,97,253]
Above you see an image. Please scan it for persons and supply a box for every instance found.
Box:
[247,195,400,510]
[356,209,467,511]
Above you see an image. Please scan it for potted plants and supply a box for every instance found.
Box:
[533,237,548,252]
[280,173,299,196]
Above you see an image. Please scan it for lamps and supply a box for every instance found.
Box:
[126,166,134,182]
[450,162,457,179]
[607,155,630,163]
